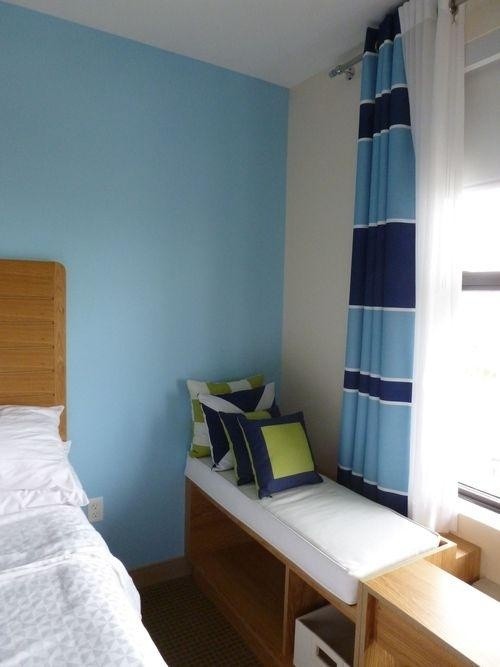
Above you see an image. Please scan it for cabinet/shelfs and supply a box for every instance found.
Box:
[184,451,500,665]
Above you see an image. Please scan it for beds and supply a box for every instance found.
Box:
[0,258,169,666]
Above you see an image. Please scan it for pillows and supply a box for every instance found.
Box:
[0,404,89,516]
[185,372,324,499]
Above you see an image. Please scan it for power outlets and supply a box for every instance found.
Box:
[87,495,103,522]
